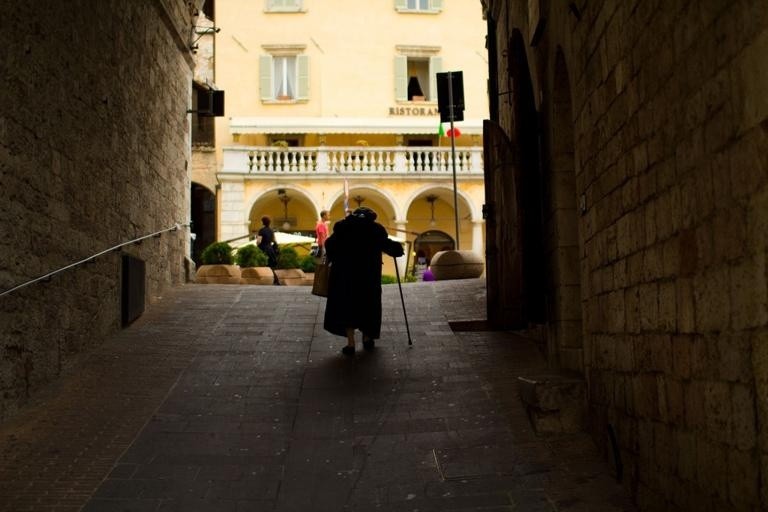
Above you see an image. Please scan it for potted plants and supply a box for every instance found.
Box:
[196,242,315,287]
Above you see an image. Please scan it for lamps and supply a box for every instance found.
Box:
[279,196,292,230]
[427,195,438,226]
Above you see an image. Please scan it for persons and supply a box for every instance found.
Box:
[255,215,275,253]
[422,258,436,282]
[315,210,331,265]
[324,206,403,355]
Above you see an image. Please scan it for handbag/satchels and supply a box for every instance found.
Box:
[311,263,331,297]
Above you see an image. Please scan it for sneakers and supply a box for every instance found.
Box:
[363,341,374,351]
[342,346,355,355]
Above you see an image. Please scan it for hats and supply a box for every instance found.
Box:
[353,207,377,219]
